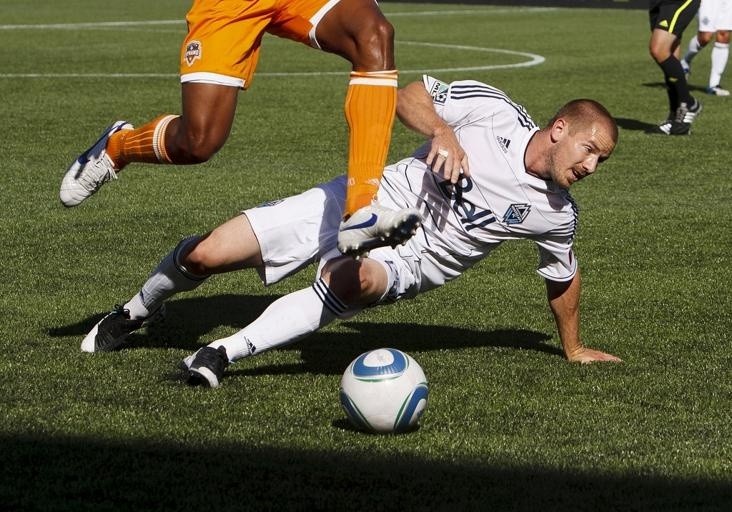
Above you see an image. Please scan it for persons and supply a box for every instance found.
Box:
[649,0,703,136]
[80,74,624,389]
[681,0,732,97]
[60,0,423,262]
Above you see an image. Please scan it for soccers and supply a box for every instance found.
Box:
[339,348,429,434]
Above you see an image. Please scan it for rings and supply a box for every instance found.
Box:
[436,150,448,158]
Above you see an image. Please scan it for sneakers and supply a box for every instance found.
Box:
[59,121,135,206]
[183,346,231,389]
[708,85,730,97]
[660,97,702,137]
[337,203,421,260]
[80,286,165,353]
